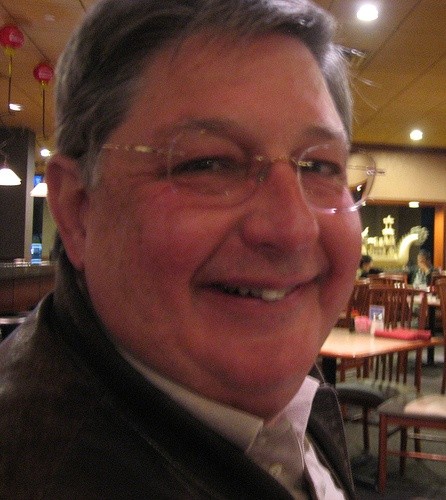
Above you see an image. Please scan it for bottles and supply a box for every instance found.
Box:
[371,312,384,334]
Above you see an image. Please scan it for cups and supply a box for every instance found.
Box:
[30,243,42,265]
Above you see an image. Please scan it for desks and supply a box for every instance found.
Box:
[320,327,444,451]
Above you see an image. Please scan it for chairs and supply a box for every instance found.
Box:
[319,269,446,494]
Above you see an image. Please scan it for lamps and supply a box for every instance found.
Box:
[0,155,22,186]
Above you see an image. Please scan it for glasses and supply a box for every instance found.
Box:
[87,140,382,213]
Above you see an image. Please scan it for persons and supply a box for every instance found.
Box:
[1,0,384,500]
[412,250,437,289]
[354,256,372,282]
